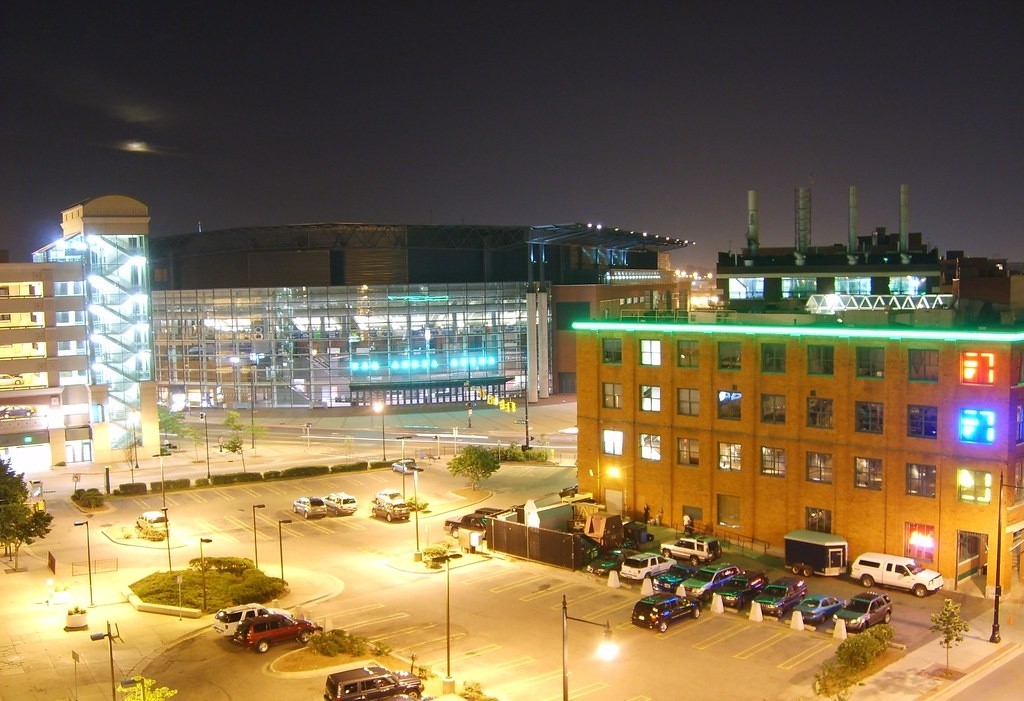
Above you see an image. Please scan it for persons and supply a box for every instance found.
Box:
[164,438,172,449]
[684,512,690,532]
[643,504,650,524]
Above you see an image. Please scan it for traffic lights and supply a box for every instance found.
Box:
[505,402,510,413]
[499,399,505,409]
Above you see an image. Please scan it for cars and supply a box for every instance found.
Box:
[0,404,36,419]
[136,511,170,532]
[372,489,411,523]
[0,373,24,387]
[392,459,419,475]
[445,507,505,541]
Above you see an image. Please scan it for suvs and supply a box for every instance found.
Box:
[324,664,424,701]
[849,551,945,599]
[321,492,358,516]
[212,602,293,638]
[234,613,315,654]
[292,496,327,520]
[586,532,894,636]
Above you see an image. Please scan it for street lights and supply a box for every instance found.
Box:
[229,355,254,449]
[374,402,386,461]
[253,504,266,568]
[409,466,424,551]
[397,435,412,501]
[432,554,463,680]
[74,520,93,606]
[201,538,212,610]
[562,594,617,701]
[90,621,116,701]
[279,520,292,580]
[161,507,171,571]
[199,412,212,482]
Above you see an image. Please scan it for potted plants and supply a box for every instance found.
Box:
[66,606,87,627]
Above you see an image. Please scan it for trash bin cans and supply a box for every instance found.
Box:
[29,479,44,500]
[453,526,482,554]
[624,521,648,543]
